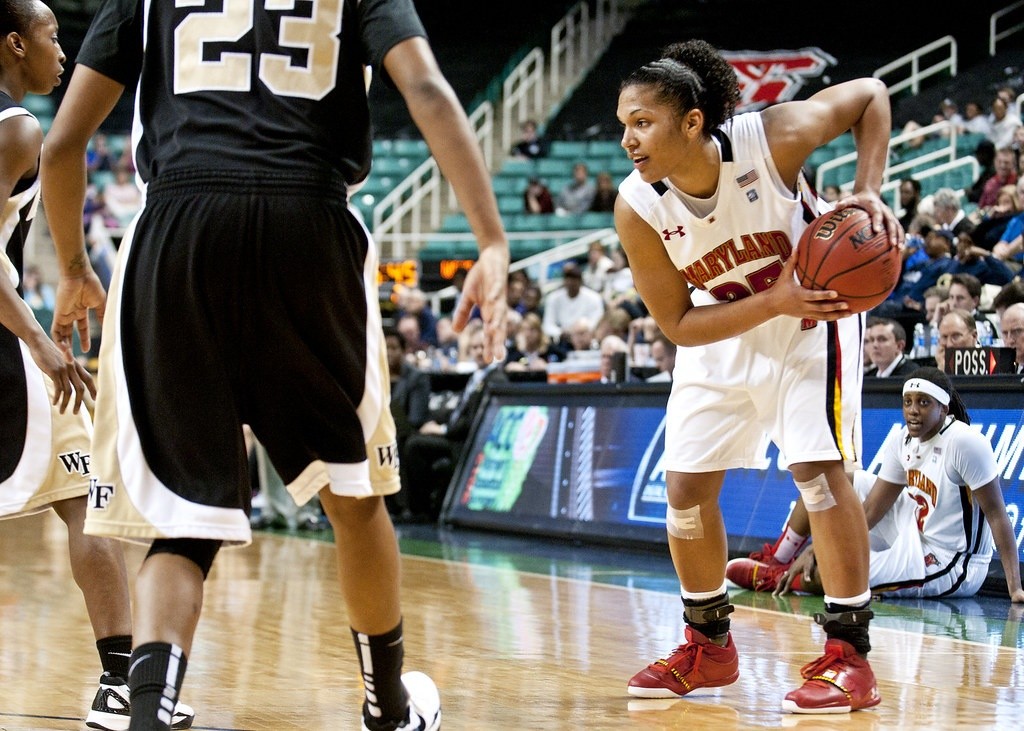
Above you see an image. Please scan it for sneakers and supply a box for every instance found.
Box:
[361,670,441,731]
[726,542,794,593]
[782,639,881,714]
[85,672,195,730]
[626,624,740,698]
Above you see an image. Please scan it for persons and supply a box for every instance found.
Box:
[823,86,1024,380]
[724,367,1024,603]
[41,0,512,730]
[377,117,679,530]
[0,0,192,731]
[21,134,143,313]
[614,41,906,714]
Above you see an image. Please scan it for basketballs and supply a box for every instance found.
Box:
[796,206,904,316]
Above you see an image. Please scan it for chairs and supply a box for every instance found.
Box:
[22,93,989,263]
